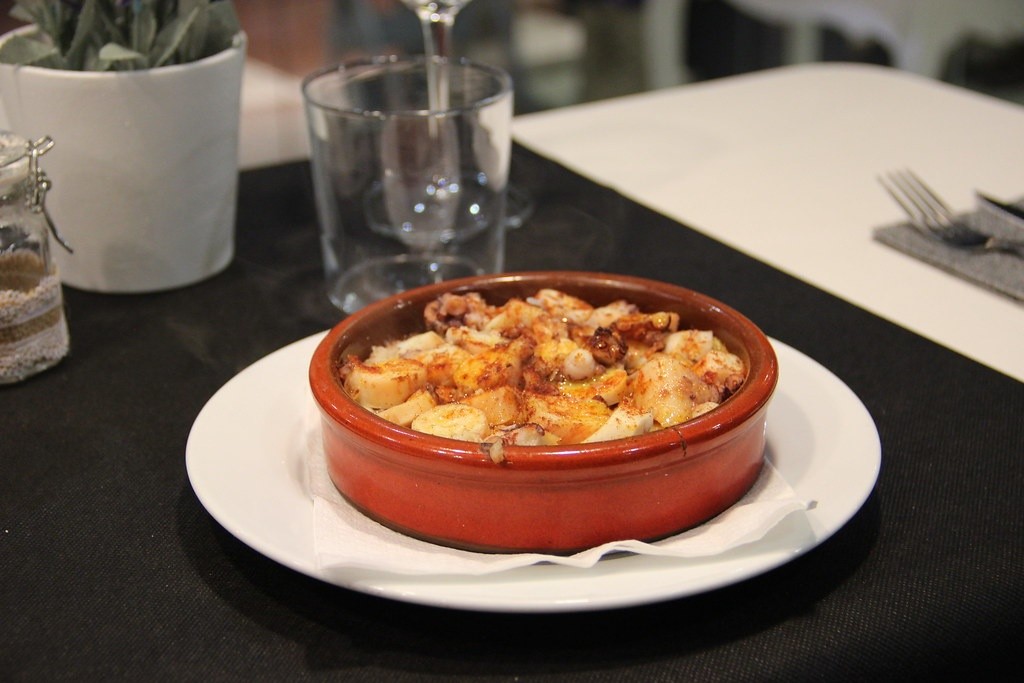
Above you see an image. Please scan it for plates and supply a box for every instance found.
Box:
[184,326,881,613]
[308,270,779,554]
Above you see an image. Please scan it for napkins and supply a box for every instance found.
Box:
[304,410,817,575]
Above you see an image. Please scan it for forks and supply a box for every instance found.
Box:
[878,165,1024,260]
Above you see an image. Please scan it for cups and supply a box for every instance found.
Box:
[301,54,515,322]
[0,22,247,294]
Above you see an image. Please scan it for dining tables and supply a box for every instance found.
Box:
[1,62,1024,683]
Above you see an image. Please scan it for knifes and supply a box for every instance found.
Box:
[973,188,1024,232]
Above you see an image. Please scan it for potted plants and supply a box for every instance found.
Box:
[0,0,248,293]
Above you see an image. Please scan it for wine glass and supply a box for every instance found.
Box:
[362,0,532,240]
